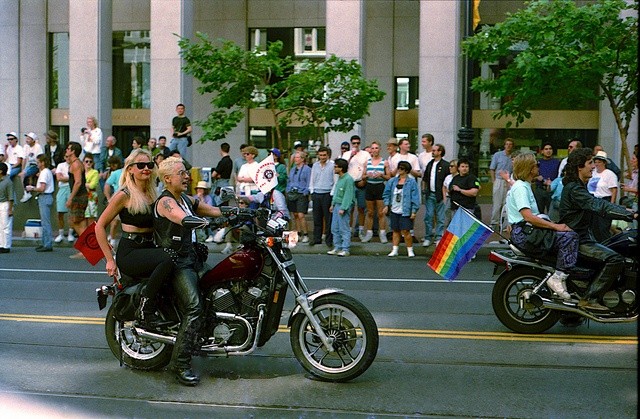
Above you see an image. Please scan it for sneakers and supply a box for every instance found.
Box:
[360,232,373,243]
[0,248,10,252]
[69,253,86,259]
[379,233,389,244]
[407,251,415,257]
[336,250,351,257]
[54,235,64,242]
[204,235,214,242]
[298,235,309,242]
[326,249,341,255]
[577,299,610,314]
[387,251,398,257]
[358,230,365,240]
[68,235,75,242]
[220,247,232,254]
[19,194,33,203]
[423,240,431,246]
[434,240,441,247]
[546,272,571,300]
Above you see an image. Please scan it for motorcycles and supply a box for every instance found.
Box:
[95,186,379,382]
[488,211,639,334]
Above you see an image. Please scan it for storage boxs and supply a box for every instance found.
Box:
[25,219,42,238]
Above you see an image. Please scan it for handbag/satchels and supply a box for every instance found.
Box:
[288,193,301,201]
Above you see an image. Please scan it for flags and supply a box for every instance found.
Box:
[74,221,113,267]
[425,207,492,282]
[249,152,278,195]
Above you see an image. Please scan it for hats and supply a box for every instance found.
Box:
[384,138,400,147]
[0,144,4,157]
[294,141,303,149]
[194,181,208,190]
[44,130,59,142]
[267,148,280,159]
[6,132,18,143]
[24,132,38,143]
[592,151,611,164]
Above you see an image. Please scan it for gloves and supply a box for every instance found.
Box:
[251,209,261,217]
[181,216,228,229]
[237,213,256,224]
[219,206,262,218]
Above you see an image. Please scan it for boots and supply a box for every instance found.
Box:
[134,296,158,334]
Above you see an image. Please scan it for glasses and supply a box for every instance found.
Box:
[449,165,454,167]
[244,153,249,156]
[129,162,155,169]
[536,161,541,168]
[85,160,92,163]
[397,166,404,170]
[6,138,16,140]
[180,170,189,176]
[341,147,348,149]
[351,142,360,145]
[238,202,245,204]
[568,146,573,149]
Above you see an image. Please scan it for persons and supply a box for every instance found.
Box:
[99,156,122,179]
[233,144,248,195]
[352,146,379,239]
[441,159,460,236]
[65,142,88,259]
[558,141,582,177]
[290,141,310,167]
[535,143,561,214]
[448,159,482,259]
[131,136,144,149]
[4,131,23,178]
[18,132,43,203]
[154,155,261,386]
[384,138,400,172]
[80,116,103,169]
[546,164,566,222]
[390,139,422,244]
[0,163,17,253]
[506,150,579,300]
[309,147,339,247]
[211,143,233,206]
[587,151,619,242]
[103,167,123,241]
[421,144,450,247]
[489,137,515,231]
[191,181,214,242]
[99,136,123,205]
[381,161,421,258]
[151,153,164,192]
[593,144,622,182]
[158,136,170,156]
[83,153,99,227]
[499,150,521,186]
[44,130,67,171]
[94,148,178,327]
[168,104,192,158]
[337,141,350,159]
[56,150,75,243]
[361,141,391,244]
[326,158,357,257]
[220,197,257,255]
[256,189,291,224]
[341,135,372,241]
[559,147,638,311]
[26,154,55,251]
[285,151,312,243]
[237,146,259,225]
[419,133,437,242]
[620,151,639,229]
[0,152,11,176]
[142,138,160,156]
[266,148,287,196]
[169,149,192,171]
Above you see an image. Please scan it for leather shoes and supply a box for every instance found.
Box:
[326,242,333,247]
[309,240,322,246]
[170,365,200,385]
[37,245,52,252]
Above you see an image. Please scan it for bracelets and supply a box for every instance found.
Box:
[380,174,383,177]
[541,178,546,184]
[506,179,512,182]
[422,190,427,194]
[31,186,34,191]
